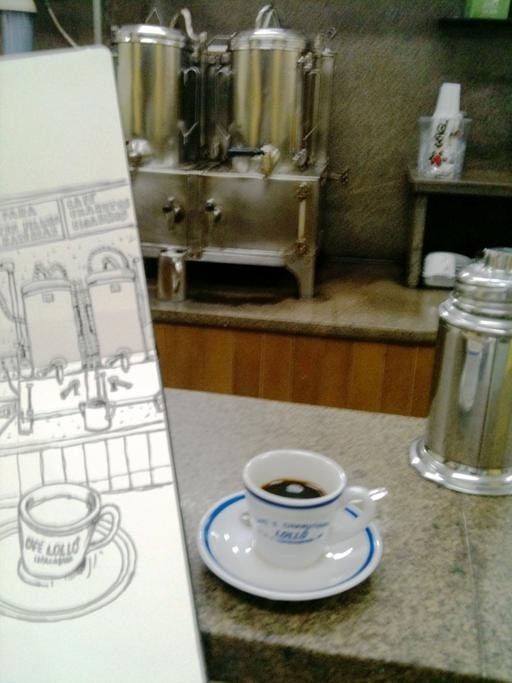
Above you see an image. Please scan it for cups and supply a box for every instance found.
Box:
[414,116,471,178]
[240,451,374,565]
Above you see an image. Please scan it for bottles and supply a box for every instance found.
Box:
[409,246,512,501]
[157,248,190,302]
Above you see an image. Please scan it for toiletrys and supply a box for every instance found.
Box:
[430,81,463,171]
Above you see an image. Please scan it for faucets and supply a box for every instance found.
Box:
[161,199,183,231]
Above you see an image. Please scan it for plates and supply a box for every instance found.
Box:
[197,489,381,601]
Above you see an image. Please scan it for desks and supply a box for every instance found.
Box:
[163,385,512,681]
